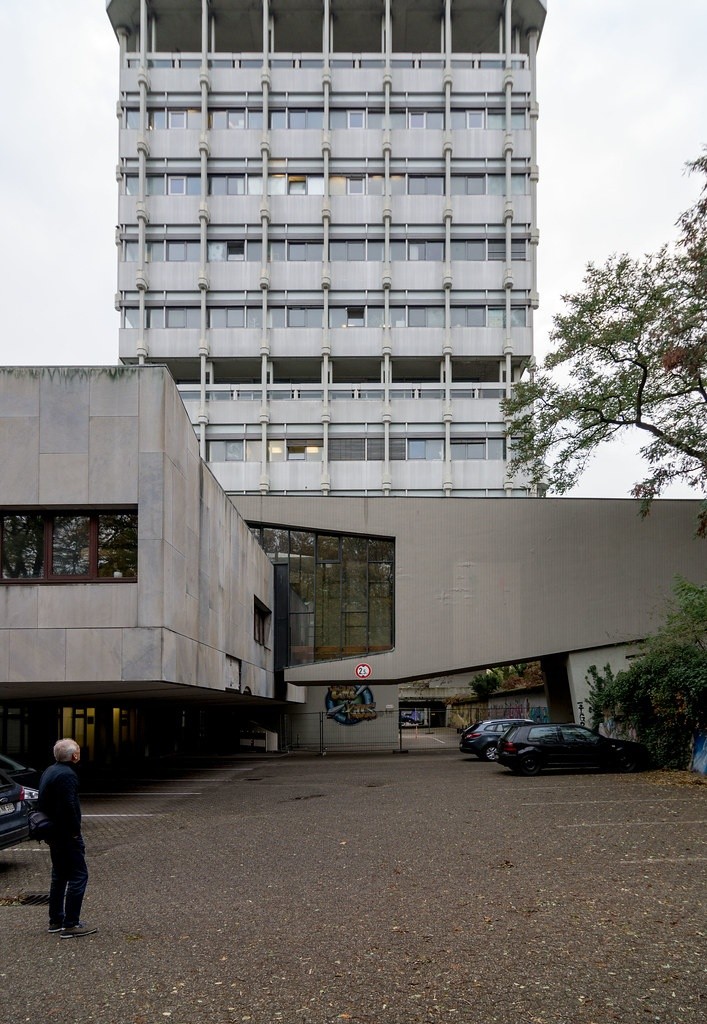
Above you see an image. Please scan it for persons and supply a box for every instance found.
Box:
[37,738,99,938]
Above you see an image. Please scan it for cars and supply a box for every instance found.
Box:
[0,752,42,851]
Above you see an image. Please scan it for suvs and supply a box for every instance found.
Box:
[458,718,541,764]
[495,720,650,778]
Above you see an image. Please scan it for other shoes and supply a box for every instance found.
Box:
[60,925,97,938]
[47,923,65,933]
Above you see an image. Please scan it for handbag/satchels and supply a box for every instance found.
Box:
[27,811,51,843]
